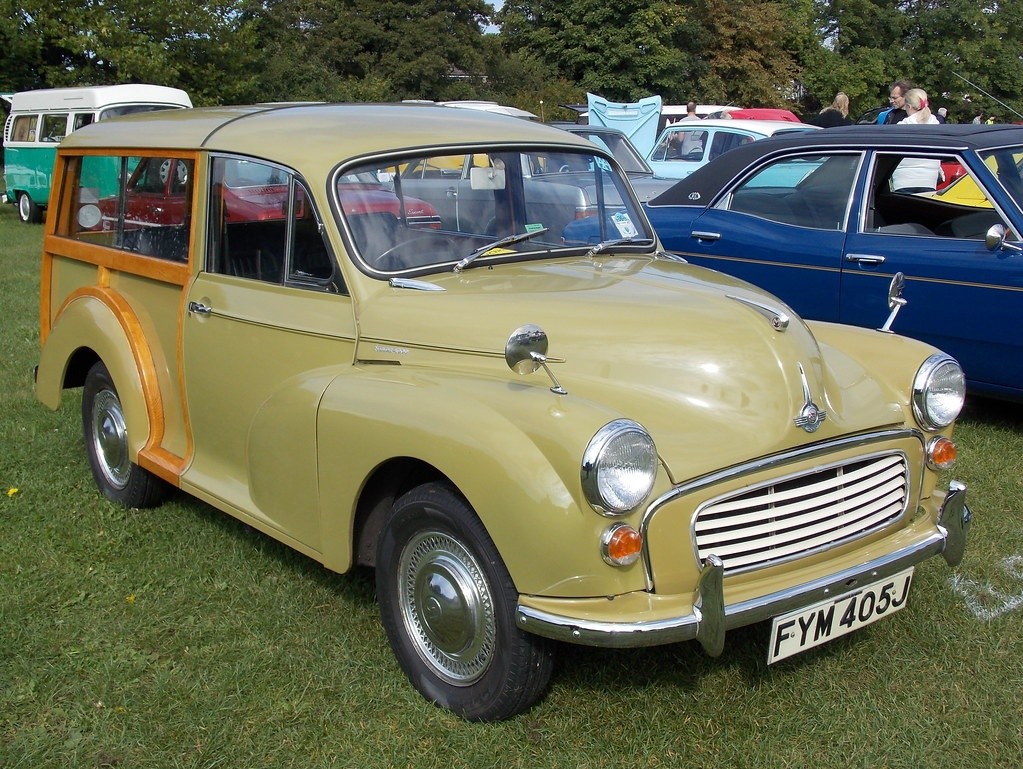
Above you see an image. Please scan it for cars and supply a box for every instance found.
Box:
[379,100,1023,242]
[560,120,1023,406]
[79,156,443,232]
[33,97,971,721]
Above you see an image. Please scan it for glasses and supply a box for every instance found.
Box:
[888,95,900,99]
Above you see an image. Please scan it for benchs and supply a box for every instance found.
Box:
[136,210,402,284]
[732,184,849,229]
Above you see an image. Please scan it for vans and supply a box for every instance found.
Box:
[0,84,194,224]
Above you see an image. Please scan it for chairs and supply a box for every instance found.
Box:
[946,210,1007,239]
[876,223,934,236]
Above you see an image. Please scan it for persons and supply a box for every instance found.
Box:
[972,111,982,124]
[884,79,911,124]
[672,101,703,160]
[806,92,851,128]
[888,88,945,195]
[984,115,996,125]
[935,108,948,124]
